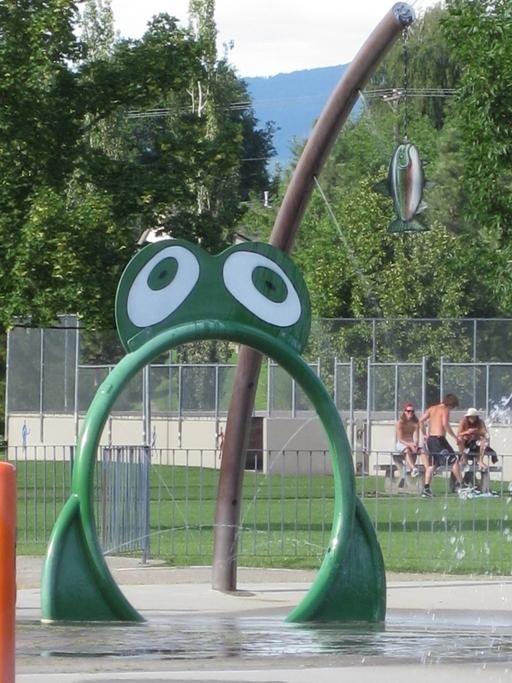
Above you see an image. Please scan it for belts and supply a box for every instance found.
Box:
[429,436,444,438]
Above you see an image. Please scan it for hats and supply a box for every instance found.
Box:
[464,408,483,416]
[405,403,413,407]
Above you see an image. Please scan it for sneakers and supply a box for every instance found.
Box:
[411,467,419,478]
[454,481,470,490]
[421,486,432,497]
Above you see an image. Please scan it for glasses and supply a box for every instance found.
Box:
[406,410,414,413]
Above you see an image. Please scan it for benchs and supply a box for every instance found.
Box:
[373,463,502,495]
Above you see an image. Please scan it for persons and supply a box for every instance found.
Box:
[396,404,429,478]
[456,406,490,469]
[417,393,474,498]
